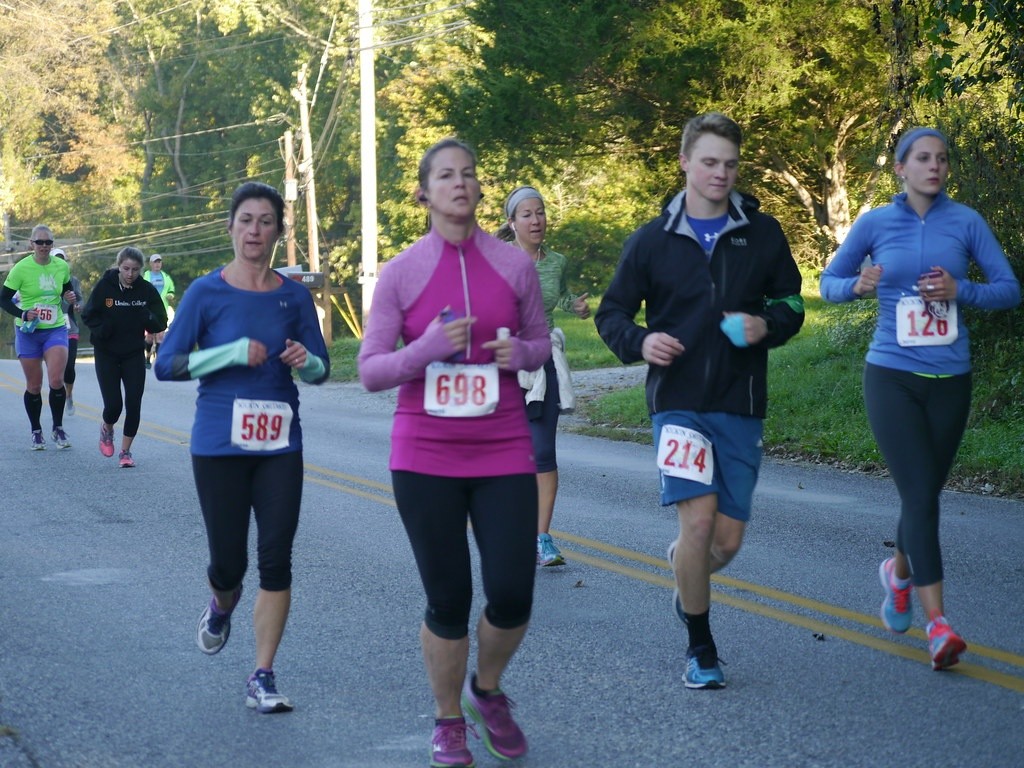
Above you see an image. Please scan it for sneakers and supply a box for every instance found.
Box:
[668,540,688,627]
[65,398,75,415]
[31,429,46,450]
[462,670,527,760]
[926,608,966,670]
[99,421,114,457]
[119,449,135,467]
[538,533,565,566]
[51,425,72,449]
[246,668,294,712]
[879,558,914,633]
[429,715,480,768]
[682,644,727,690]
[196,583,243,656]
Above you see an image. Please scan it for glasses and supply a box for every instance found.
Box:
[33,240,53,245]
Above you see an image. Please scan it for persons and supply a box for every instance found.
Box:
[143,254,175,369]
[0,225,85,450]
[494,185,591,566]
[594,114,806,689]
[356,139,552,768]
[153,182,331,713]
[819,126,1020,672]
[81,246,169,468]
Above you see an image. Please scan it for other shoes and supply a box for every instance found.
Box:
[146,355,152,369]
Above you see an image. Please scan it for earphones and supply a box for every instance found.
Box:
[913,286,920,292]
[927,284,934,290]
[479,193,484,198]
[420,195,428,201]
[511,222,516,230]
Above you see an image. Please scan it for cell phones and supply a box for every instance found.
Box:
[920,271,941,279]
[440,312,465,362]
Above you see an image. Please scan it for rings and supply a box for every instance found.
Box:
[296,359,299,363]
[926,284,935,291]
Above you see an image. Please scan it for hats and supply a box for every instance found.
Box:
[51,249,66,260]
[150,254,161,262]
